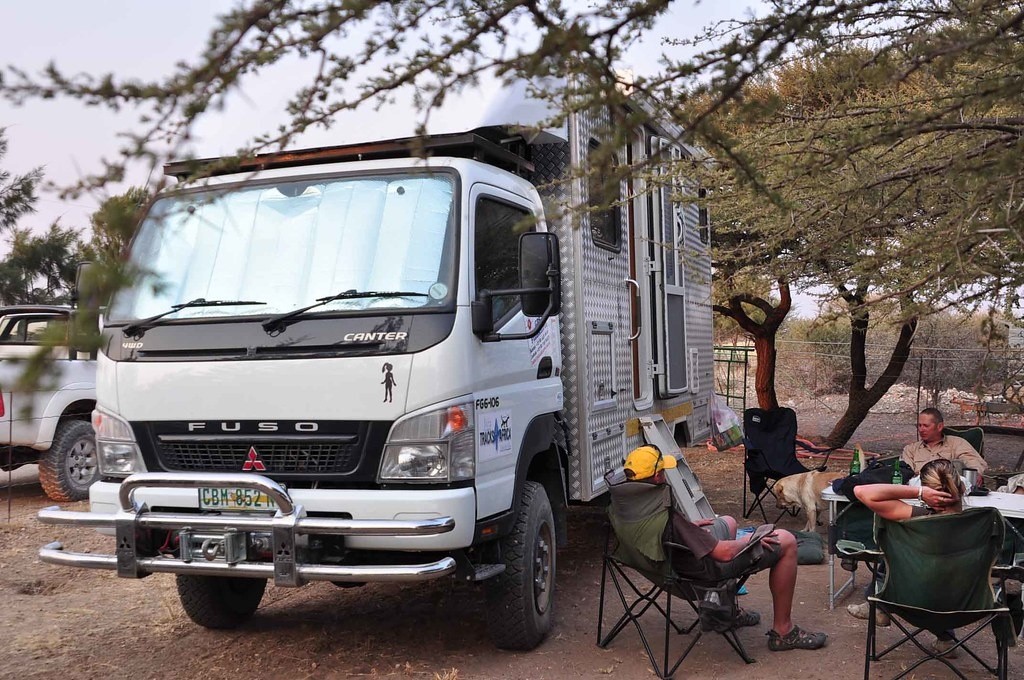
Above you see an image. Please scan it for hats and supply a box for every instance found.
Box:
[623,445,677,481]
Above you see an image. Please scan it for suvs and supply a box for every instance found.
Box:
[0,304,112,503]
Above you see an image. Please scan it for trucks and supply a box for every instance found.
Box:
[33,51,717,652]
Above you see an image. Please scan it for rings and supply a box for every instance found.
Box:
[941,497,943,501]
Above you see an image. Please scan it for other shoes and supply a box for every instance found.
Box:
[846,600,891,627]
[931,637,959,659]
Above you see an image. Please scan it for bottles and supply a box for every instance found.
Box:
[891,459,903,485]
[962,468,979,493]
[850,448,861,477]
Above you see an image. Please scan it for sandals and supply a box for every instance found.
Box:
[765,625,828,651]
[736,605,760,628]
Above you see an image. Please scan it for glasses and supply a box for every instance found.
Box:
[920,459,955,480]
[640,444,662,476]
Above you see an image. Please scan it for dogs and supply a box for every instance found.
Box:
[772,469,849,534]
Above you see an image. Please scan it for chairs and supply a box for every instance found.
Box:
[596,406,1024,680]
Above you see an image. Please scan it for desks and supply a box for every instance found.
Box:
[821,483,1024,612]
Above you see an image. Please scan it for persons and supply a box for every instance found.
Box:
[623,444,826,652]
[847,458,966,659]
[898,408,989,487]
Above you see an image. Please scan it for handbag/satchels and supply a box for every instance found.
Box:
[706,388,745,453]
[832,458,915,511]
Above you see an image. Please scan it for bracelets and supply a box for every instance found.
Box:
[918,485,922,501]
